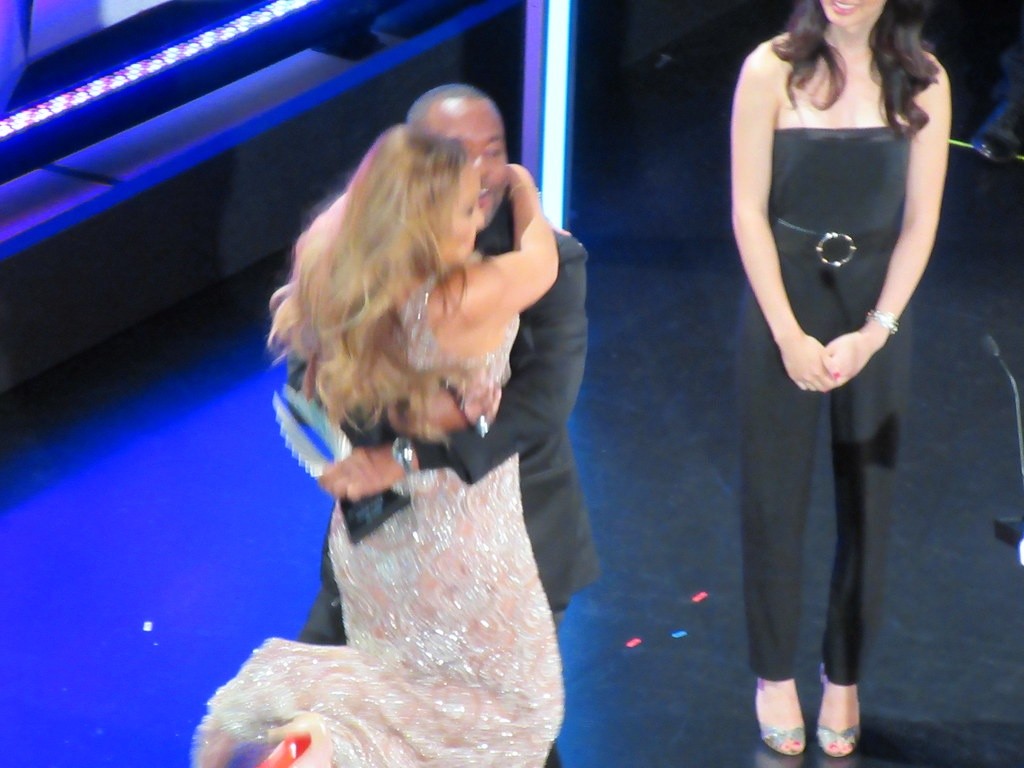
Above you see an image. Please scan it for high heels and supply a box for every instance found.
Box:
[816,662,861,758]
[755,677,806,756]
[254,711,333,768]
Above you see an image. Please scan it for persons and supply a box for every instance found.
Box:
[732,0,952,756]
[189,83,601,767]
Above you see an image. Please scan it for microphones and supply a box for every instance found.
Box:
[981,334,1024,479]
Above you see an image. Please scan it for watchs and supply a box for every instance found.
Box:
[866,307,899,338]
[392,431,414,476]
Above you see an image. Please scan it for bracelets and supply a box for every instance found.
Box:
[504,181,541,202]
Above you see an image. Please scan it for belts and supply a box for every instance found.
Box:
[776,219,890,267]
